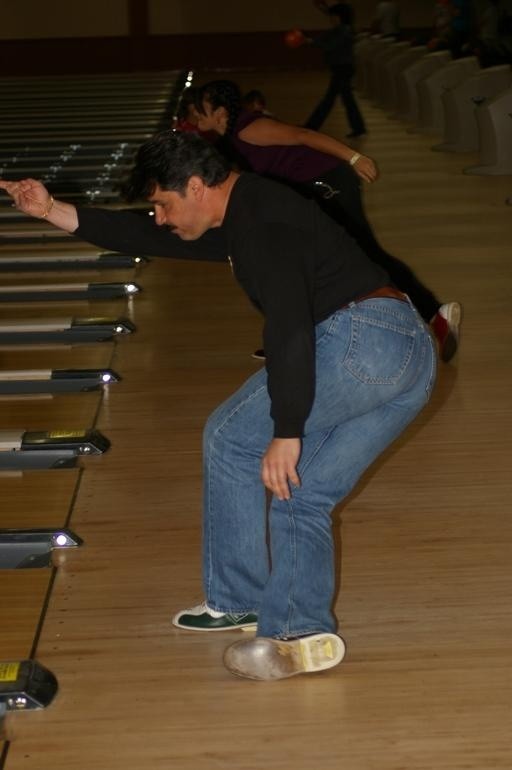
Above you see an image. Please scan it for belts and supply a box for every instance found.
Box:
[341,286,411,310]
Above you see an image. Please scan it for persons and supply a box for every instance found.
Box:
[298,2,367,138]
[187,80,460,363]
[425,0,512,69]
[0,128,435,681]
[369,0,402,40]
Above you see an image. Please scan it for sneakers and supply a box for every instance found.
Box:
[428,302,463,363]
[346,127,367,139]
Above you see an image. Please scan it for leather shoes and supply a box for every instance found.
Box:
[222,631,346,682]
[172,600,261,633]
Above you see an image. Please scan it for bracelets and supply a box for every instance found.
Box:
[39,196,54,219]
[350,152,360,165]
